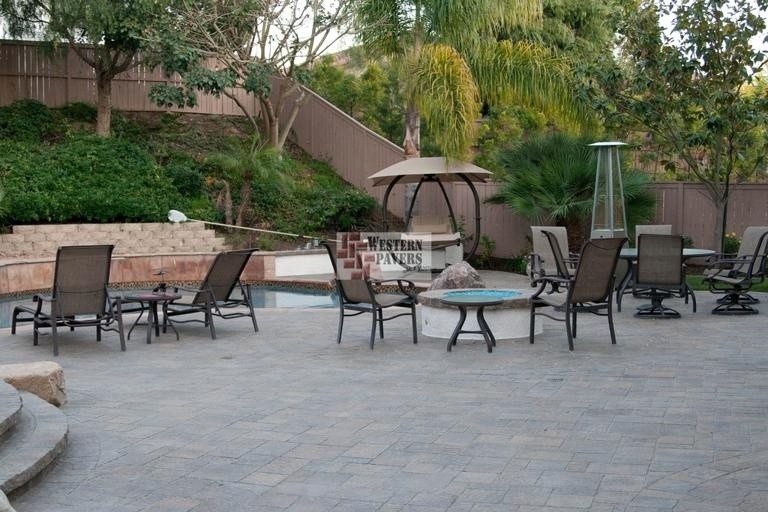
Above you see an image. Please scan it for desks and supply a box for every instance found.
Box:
[113,290,183,344]
[438,293,505,355]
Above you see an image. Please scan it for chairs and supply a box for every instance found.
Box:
[399,212,460,251]
[9,242,119,359]
[526,220,767,323]
[528,236,630,353]
[159,249,261,343]
[322,236,420,349]
